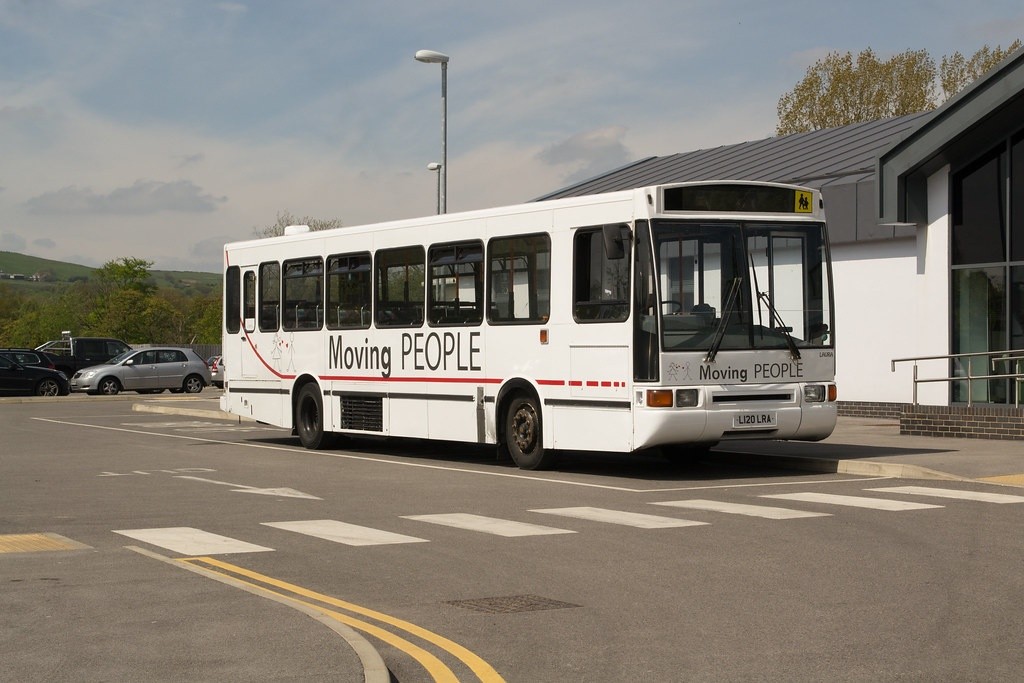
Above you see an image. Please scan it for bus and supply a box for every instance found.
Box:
[218,178,838,472]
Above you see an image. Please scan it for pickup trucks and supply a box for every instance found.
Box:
[21,336,173,395]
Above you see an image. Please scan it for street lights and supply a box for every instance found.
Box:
[427,162,442,214]
[414,49,449,214]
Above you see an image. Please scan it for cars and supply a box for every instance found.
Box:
[0,347,71,398]
[206,355,224,389]
[71,346,212,396]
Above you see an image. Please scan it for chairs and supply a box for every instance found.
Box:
[143,356,152,363]
[263,298,498,329]
